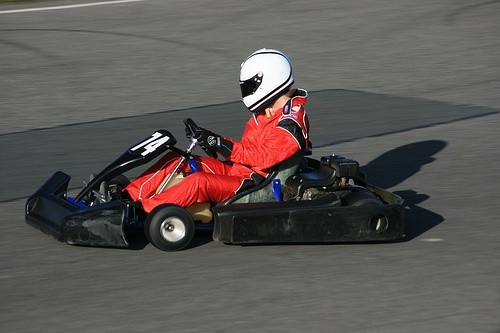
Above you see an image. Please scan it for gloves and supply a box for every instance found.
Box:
[185,127,222,143]
[195,127,233,158]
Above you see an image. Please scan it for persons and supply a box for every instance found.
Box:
[88,45,311,220]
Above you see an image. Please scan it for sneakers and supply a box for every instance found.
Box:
[98,182,142,229]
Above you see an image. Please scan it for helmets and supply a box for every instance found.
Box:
[238,47,294,113]
